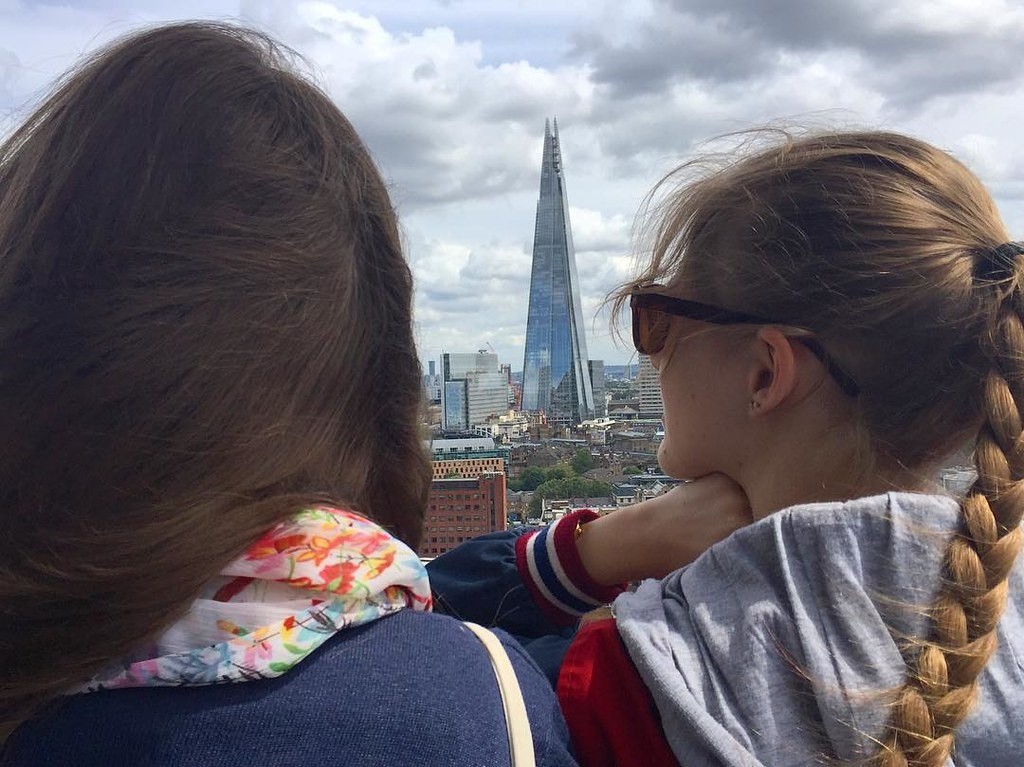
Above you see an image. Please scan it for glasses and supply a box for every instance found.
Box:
[624,279,864,405]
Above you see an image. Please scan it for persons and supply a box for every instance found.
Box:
[0,21,582,767]
[420,130,1024,767]
